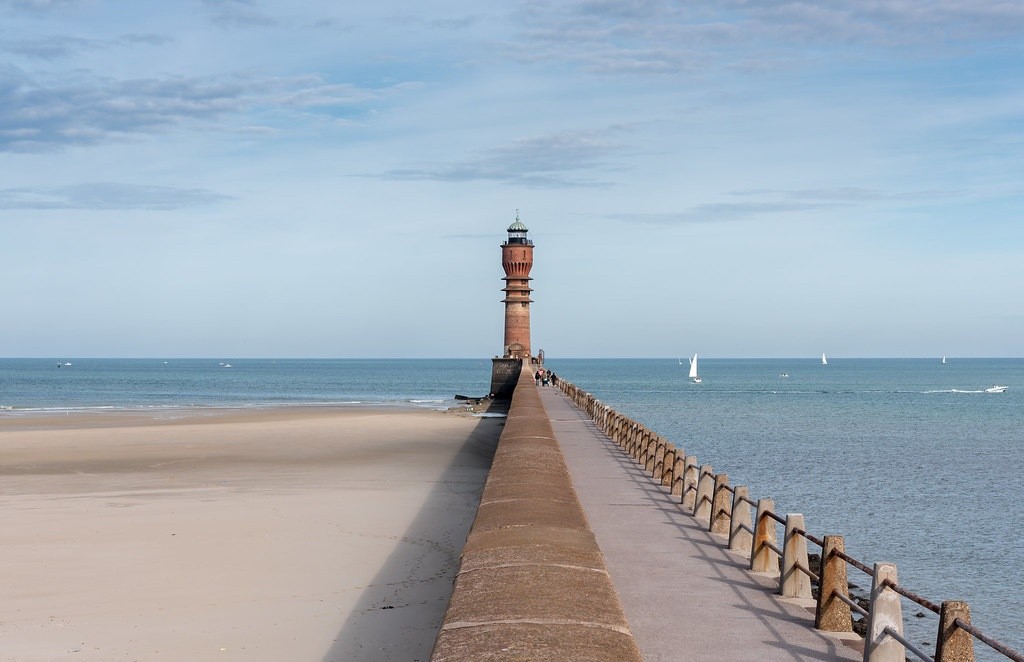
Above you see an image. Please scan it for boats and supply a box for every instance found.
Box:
[986,384,1008,393]
[778,372,789,378]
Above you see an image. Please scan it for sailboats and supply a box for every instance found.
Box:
[822,353,827,365]
[688,353,702,383]
[941,356,946,364]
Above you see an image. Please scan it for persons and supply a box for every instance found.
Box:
[550,373,556,386]
[539,368,551,387]
[532,356,538,364]
[535,371,540,386]
